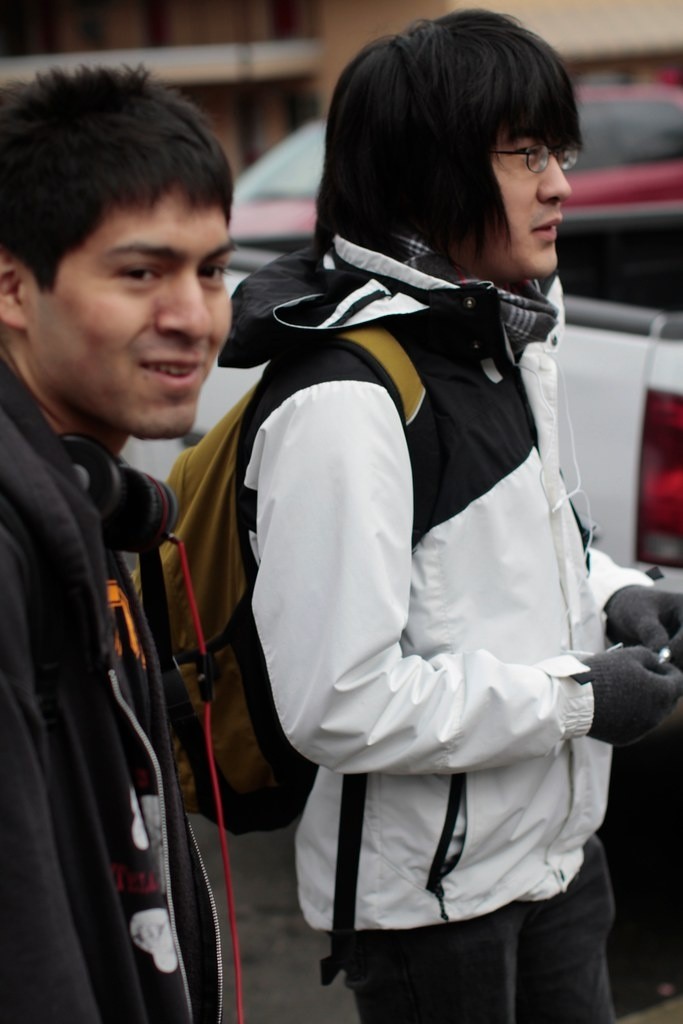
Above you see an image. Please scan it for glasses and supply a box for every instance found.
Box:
[491,140,579,173]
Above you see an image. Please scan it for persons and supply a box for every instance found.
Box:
[219,10,683,1024]
[0,66,234,1024]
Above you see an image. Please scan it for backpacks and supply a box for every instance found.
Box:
[130,325,440,836]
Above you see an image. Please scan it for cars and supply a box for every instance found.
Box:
[230,66,683,236]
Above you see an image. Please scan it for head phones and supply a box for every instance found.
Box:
[58,432,178,553]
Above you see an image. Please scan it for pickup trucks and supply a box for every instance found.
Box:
[122,205,683,596]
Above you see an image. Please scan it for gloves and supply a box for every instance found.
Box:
[569,645,683,745]
[604,585,683,671]
